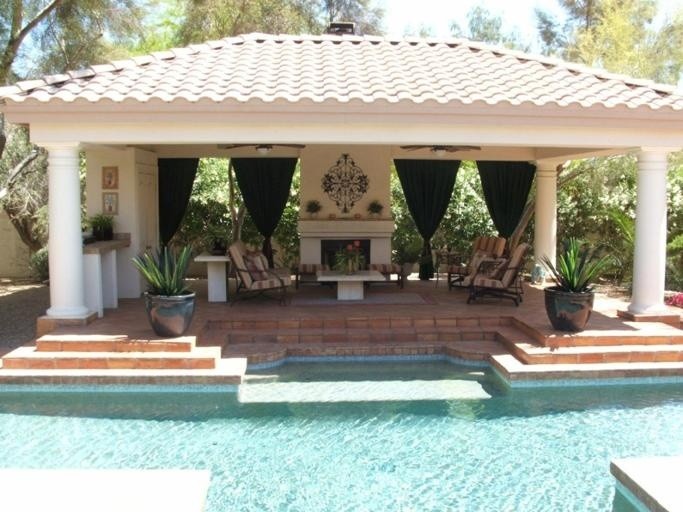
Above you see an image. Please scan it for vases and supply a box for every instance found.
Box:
[100,165,119,215]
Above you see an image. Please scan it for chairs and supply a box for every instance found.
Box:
[447,234,529,307]
[226,239,290,306]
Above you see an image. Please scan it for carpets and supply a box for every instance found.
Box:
[293,285,437,306]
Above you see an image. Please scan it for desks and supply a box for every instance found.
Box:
[434,251,464,289]
[192,252,230,304]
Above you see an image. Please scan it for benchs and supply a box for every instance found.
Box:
[290,262,405,289]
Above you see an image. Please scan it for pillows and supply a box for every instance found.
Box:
[242,251,269,281]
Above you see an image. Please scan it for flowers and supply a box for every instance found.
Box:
[333,239,366,272]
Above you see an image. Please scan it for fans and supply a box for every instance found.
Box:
[398,145,481,155]
[223,145,305,153]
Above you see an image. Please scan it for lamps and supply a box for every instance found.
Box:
[254,145,271,154]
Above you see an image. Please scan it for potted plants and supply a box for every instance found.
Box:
[89,212,113,242]
[537,236,619,332]
[132,242,197,336]
[306,199,322,219]
[366,200,383,219]
[203,222,229,255]
